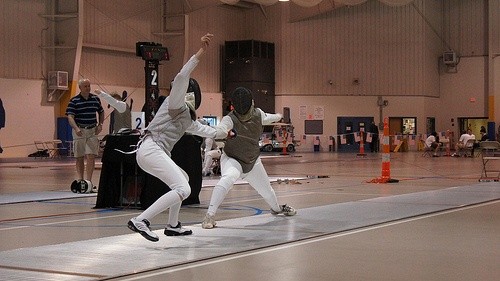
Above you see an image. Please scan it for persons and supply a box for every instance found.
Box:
[201,137,221,175]
[480,126,486,134]
[424,132,442,157]
[442,129,452,156]
[467,129,475,138]
[453,129,472,156]
[467,129,488,157]
[94,89,132,134]
[468,123,472,131]
[65,79,105,188]
[368,121,379,152]
[202,87,296,229]
[127,32,235,240]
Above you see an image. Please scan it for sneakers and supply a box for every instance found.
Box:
[271,203,297,216]
[127,217,159,242]
[201,213,216,228]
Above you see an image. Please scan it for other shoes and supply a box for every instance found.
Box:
[164,221,192,236]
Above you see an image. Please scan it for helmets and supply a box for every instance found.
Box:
[185,78,202,110]
[231,87,254,115]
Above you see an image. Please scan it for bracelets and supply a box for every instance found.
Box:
[99,124,103,126]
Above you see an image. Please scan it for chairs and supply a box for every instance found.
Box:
[65,141,74,158]
[475,139,489,158]
[53,139,67,159]
[34,141,47,160]
[458,139,476,158]
[44,141,59,159]
[418,140,433,159]
[478,141,500,182]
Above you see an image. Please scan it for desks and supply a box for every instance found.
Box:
[91,134,203,210]
